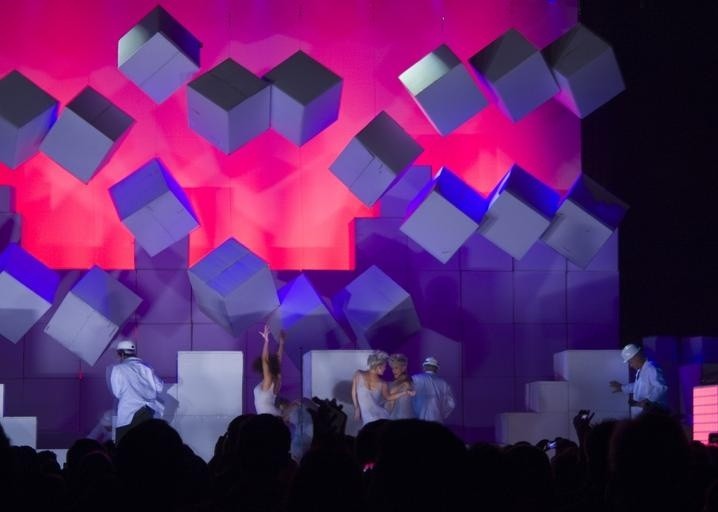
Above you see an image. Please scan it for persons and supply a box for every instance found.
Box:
[1,396,718,512]
[407,355,457,427]
[380,352,416,419]
[350,349,417,427]
[252,322,287,418]
[106,339,166,437]
[608,343,670,418]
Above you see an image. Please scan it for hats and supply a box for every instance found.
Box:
[620,342,641,364]
[115,340,136,351]
[422,355,440,368]
